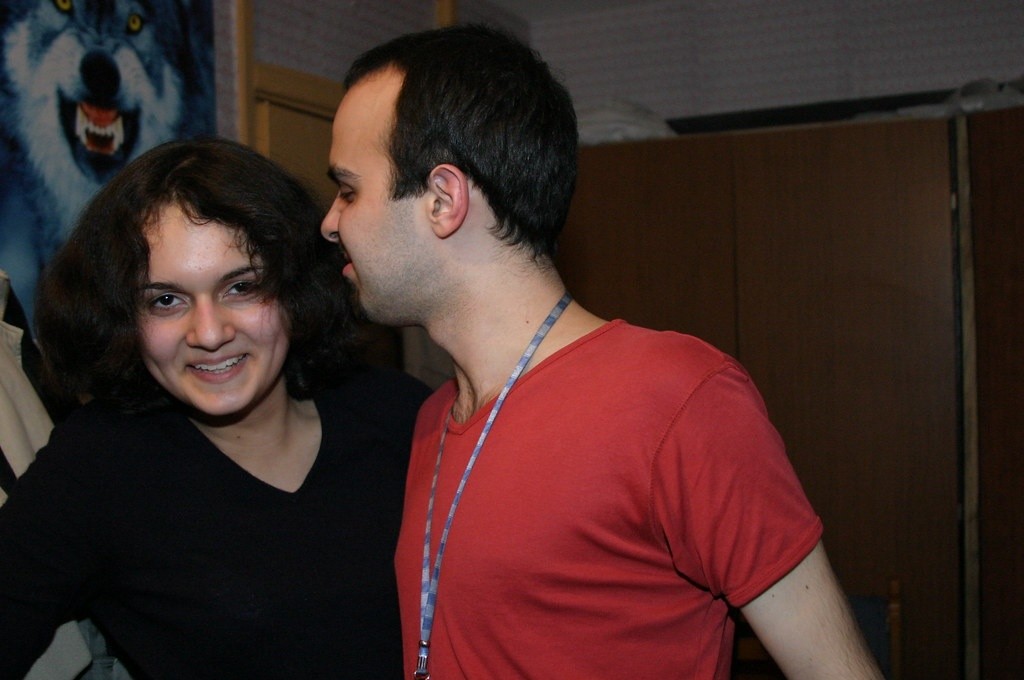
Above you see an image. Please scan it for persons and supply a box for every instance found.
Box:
[0,136,433,680]
[321,24,885,680]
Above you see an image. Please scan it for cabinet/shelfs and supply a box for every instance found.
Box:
[555,108,1024,679]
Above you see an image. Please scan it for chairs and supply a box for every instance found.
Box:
[734,576,903,680]
